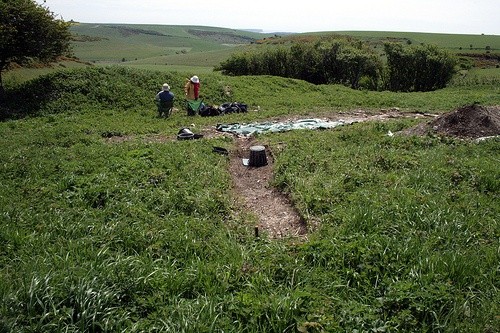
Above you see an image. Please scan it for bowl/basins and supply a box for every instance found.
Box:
[179,133,194,140]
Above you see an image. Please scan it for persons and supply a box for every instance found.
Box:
[185,75,201,115]
[154,83,174,118]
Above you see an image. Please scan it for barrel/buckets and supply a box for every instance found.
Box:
[248,146,268,168]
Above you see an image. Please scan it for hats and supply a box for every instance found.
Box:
[162,83,170,90]
[190,75,199,83]
